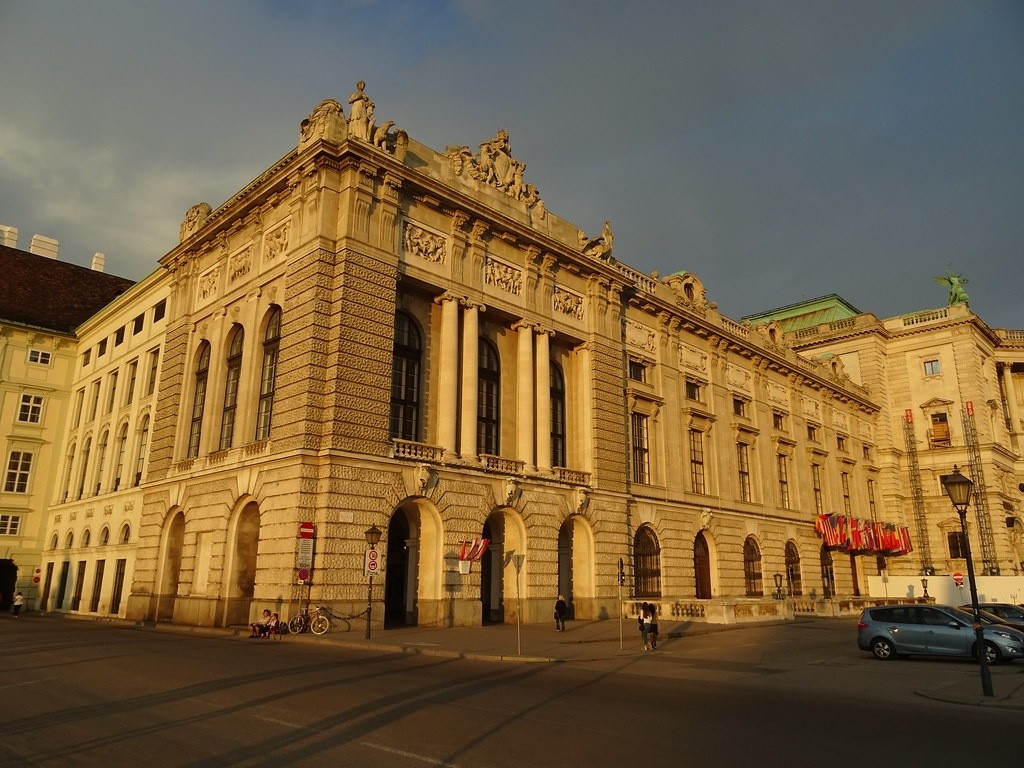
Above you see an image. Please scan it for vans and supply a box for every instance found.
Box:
[857,603,1024,666]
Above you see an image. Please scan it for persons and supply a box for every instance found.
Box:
[348,80,370,143]
[554,595,567,633]
[638,602,659,651]
[250,610,279,639]
[13,592,24,619]
[602,220,614,265]
[446,128,539,206]
[945,262,968,304]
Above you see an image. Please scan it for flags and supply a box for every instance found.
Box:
[815,512,913,556]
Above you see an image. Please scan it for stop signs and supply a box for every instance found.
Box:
[953,573,963,582]
[300,523,315,539]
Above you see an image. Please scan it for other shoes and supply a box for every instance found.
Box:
[262,635,269,639]
[555,630,560,632]
[562,629,567,632]
[652,644,660,651]
[248,635,261,638]
[641,645,649,650]
[12,616,17,618]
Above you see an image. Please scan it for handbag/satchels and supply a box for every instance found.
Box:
[638,622,645,631]
[554,609,558,620]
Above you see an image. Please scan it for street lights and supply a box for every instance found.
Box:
[364,523,382,640]
[941,464,994,696]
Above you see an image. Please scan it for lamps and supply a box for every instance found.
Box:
[772,570,785,600]
[921,576,929,598]
